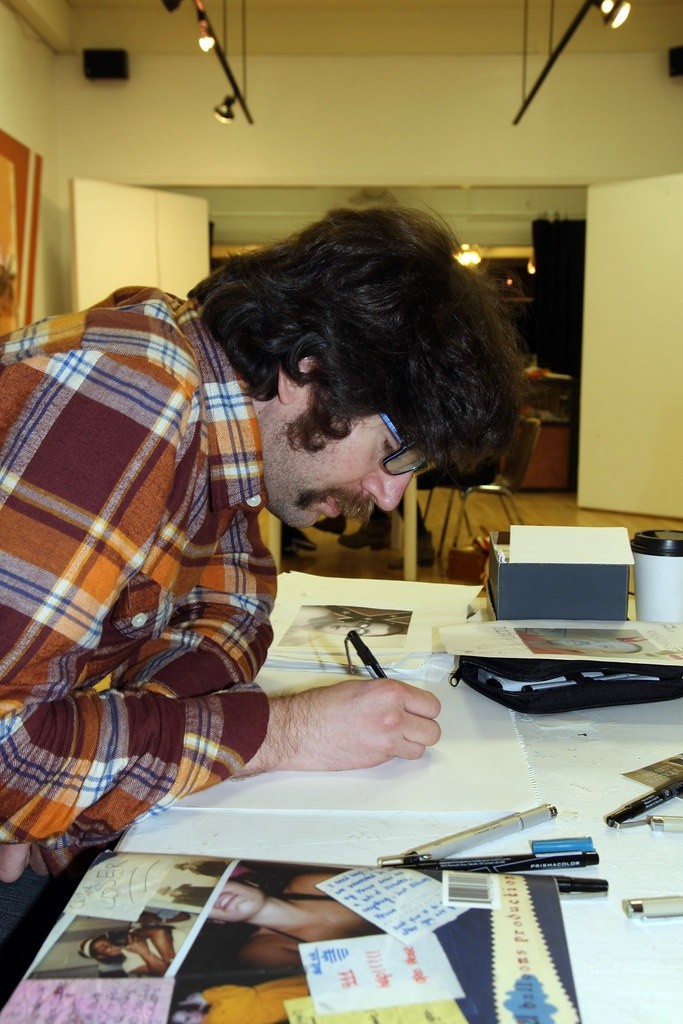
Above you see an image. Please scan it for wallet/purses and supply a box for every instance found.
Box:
[449,654,682,716]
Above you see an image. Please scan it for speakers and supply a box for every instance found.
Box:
[83,48,127,79]
[669,45,683,77]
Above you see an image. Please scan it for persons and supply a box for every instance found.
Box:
[80,911,198,975]
[517,628,642,656]
[169,976,309,1024]
[0,205,520,941]
[209,873,369,969]
[156,859,225,906]
[280,606,402,645]
[280,495,436,570]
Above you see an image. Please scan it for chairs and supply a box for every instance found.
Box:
[424,417,542,556]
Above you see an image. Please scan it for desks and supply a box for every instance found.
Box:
[1,598,683,1023]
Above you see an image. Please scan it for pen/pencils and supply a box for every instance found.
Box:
[646,814,683,834]
[344,629,388,681]
[605,776,683,830]
[611,675,660,682]
[377,801,611,898]
[623,892,683,920]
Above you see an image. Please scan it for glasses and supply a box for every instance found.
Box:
[379,411,437,482]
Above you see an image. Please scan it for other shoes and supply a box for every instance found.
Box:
[386,532,436,571]
[313,514,346,535]
[336,520,392,552]
[284,530,317,554]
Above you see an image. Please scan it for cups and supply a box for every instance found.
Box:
[630,529,683,622]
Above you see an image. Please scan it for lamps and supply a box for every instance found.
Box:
[594,0,631,29]
[197,8,215,53]
[214,93,237,124]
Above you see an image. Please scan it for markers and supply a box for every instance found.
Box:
[487,673,639,692]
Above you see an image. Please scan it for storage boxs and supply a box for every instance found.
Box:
[485,530,630,622]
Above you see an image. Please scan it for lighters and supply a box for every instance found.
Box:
[645,817,683,834]
[622,894,683,920]
